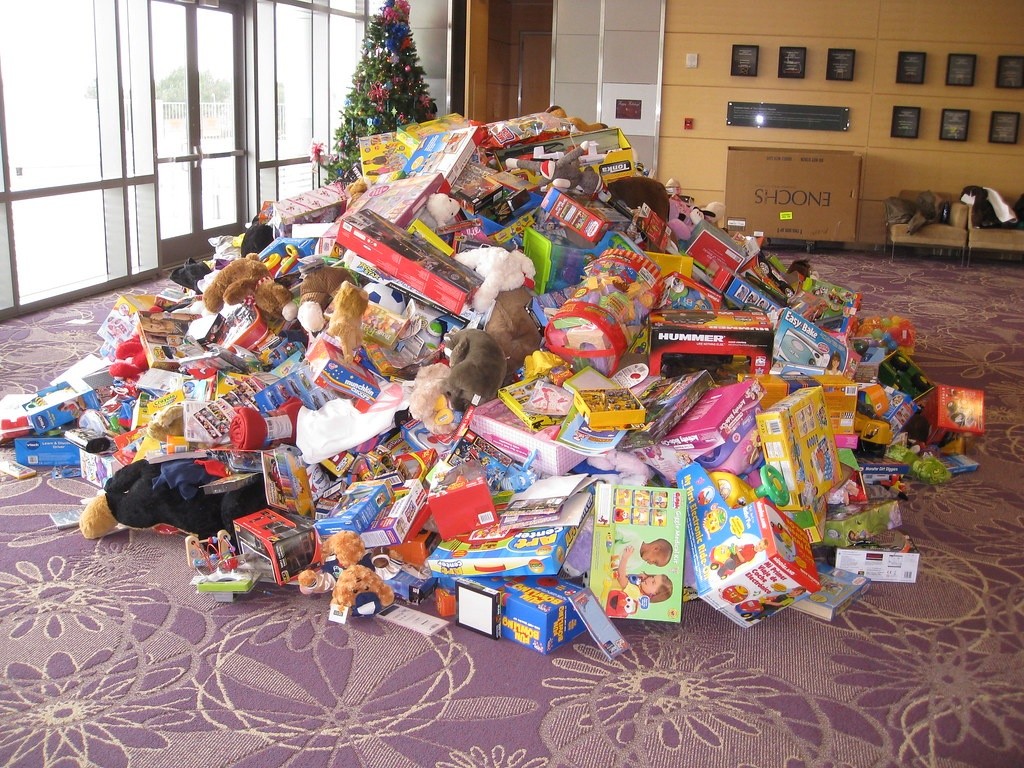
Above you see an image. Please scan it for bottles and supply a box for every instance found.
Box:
[940,200,950,223]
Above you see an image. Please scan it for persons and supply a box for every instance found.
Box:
[612,523,672,603]
[363,151,394,176]
[140,311,202,345]
[826,352,843,375]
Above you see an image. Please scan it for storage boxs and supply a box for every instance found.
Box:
[0,112,985,654]
[724,146,862,243]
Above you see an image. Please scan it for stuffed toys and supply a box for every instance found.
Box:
[327,280,370,365]
[606,176,670,222]
[485,288,542,376]
[298,529,394,617]
[546,105,608,132]
[588,450,656,486]
[409,362,462,434]
[109,334,149,380]
[204,253,291,320]
[80,458,273,556]
[669,196,726,241]
[505,140,603,193]
[885,443,951,483]
[240,213,274,258]
[453,246,536,314]
[282,257,358,332]
[446,327,507,411]
[347,177,368,210]
[146,404,184,442]
[418,193,460,229]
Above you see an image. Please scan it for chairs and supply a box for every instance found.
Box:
[883,189,1024,268]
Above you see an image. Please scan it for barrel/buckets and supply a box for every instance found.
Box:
[544,249,667,377]
